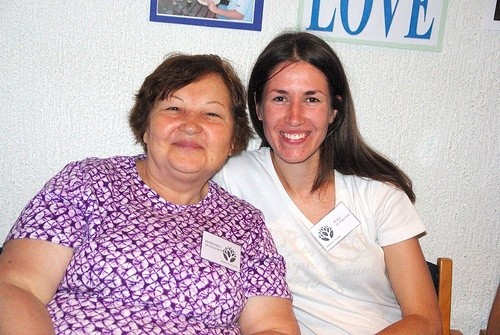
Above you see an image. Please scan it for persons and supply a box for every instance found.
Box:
[209,30,443,335]
[207,0,254,23]
[1,53,302,335]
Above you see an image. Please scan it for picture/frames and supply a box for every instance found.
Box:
[150,0,263,32]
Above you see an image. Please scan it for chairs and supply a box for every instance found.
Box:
[420,257,464,335]
